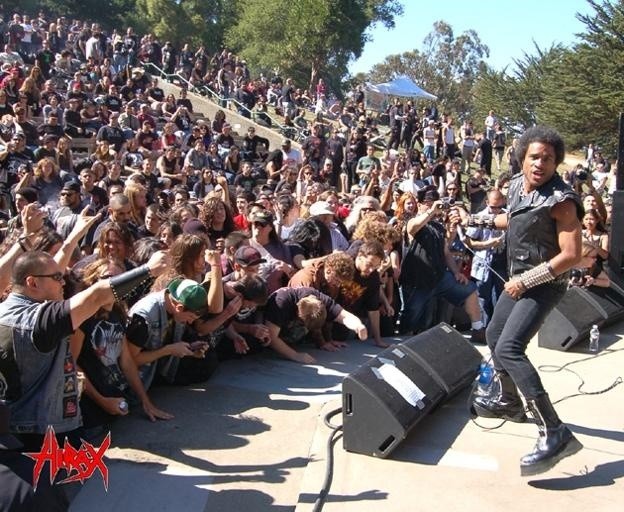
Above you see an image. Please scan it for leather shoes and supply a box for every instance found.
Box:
[473,396,527,425]
[520,423,583,475]
[471,328,486,344]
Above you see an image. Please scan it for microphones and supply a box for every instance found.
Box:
[450,210,467,241]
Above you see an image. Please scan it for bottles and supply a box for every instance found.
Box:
[477,361,494,396]
[589,325,599,352]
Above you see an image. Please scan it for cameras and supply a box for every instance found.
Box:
[442,197,456,209]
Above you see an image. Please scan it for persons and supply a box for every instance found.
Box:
[120,78,139,102]
[446,157,461,188]
[506,138,522,176]
[136,203,165,237]
[211,52,272,127]
[330,86,385,149]
[0,252,173,512]
[95,112,130,158]
[234,162,259,195]
[0,13,10,52]
[208,141,225,177]
[41,79,62,106]
[496,173,512,195]
[69,259,173,429]
[0,43,25,77]
[0,133,34,219]
[19,14,33,55]
[221,230,251,275]
[570,244,611,288]
[388,192,418,227]
[98,101,112,125]
[158,188,175,208]
[493,124,506,170]
[30,66,45,90]
[466,189,508,299]
[31,19,45,52]
[144,78,165,110]
[608,168,616,197]
[287,252,354,352]
[169,206,195,235]
[222,244,271,347]
[302,124,328,181]
[92,194,140,252]
[51,17,122,64]
[485,110,497,142]
[91,140,114,161]
[211,110,227,139]
[42,94,65,129]
[33,231,63,257]
[196,119,212,150]
[97,180,125,215]
[34,133,60,166]
[14,107,39,148]
[343,195,380,239]
[346,242,390,348]
[265,149,288,185]
[37,111,65,138]
[461,120,475,175]
[12,94,32,122]
[273,194,305,242]
[318,159,338,190]
[135,120,159,157]
[249,209,292,265]
[0,68,24,97]
[52,181,94,256]
[169,104,195,134]
[308,201,353,258]
[4,78,20,107]
[378,147,448,203]
[161,122,184,153]
[0,89,15,119]
[247,203,266,222]
[175,190,190,207]
[224,145,242,184]
[581,209,609,269]
[561,170,572,187]
[444,182,463,201]
[124,182,147,224]
[36,9,49,32]
[128,159,160,207]
[57,138,73,173]
[7,187,56,247]
[374,229,397,283]
[140,34,216,98]
[34,159,63,204]
[280,139,302,182]
[388,97,408,150]
[283,217,326,269]
[78,168,109,215]
[55,49,127,100]
[356,145,381,197]
[590,151,604,172]
[193,167,216,200]
[389,212,415,280]
[10,163,39,214]
[400,186,487,343]
[403,101,456,158]
[450,126,582,468]
[19,77,42,116]
[123,26,138,68]
[265,286,368,365]
[232,191,257,231]
[137,104,156,130]
[585,142,595,162]
[177,88,194,113]
[327,127,344,175]
[184,216,206,238]
[344,129,361,194]
[380,279,399,317]
[261,164,322,209]
[318,189,349,239]
[118,104,137,139]
[71,221,138,295]
[104,84,123,113]
[1,113,16,150]
[157,223,182,246]
[35,40,56,81]
[583,193,598,212]
[198,197,237,253]
[161,94,177,122]
[79,100,102,132]
[182,126,203,159]
[7,16,25,52]
[148,234,223,315]
[62,98,93,138]
[127,277,210,394]
[179,273,268,385]
[120,138,152,174]
[477,131,493,178]
[133,71,150,91]
[126,89,149,114]
[91,160,106,178]
[590,161,608,197]
[242,127,270,162]
[214,126,235,159]
[98,161,125,191]
[153,146,187,188]
[273,78,328,140]
[571,163,586,185]
[182,139,209,176]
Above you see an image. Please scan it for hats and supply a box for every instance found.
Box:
[252,208,273,224]
[282,140,292,147]
[167,145,176,153]
[74,82,79,86]
[109,112,119,119]
[429,120,438,126]
[417,189,440,203]
[159,189,175,202]
[233,246,267,266]
[274,179,286,194]
[408,101,416,107]
[309,200,335,216]
[396,101,402,107]
[183,216,207,234]
[222,122,232,129]
[49,110,59,117]
[15,187,40,204]
[168,277,208,310]
[68,97,80,104]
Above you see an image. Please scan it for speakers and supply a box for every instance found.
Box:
[398,322,485,409]
[578,285,624,326]
[538,287,607,352]
[342,344,448,460]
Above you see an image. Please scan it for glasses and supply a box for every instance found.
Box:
[253,221,269,228]
[448,187,458,191]
[303,171,314,176]
[489,205,504,210]
[361,207,377,212]
[59,190,75,198]
[28,273,65,281]
[204,170,213,174]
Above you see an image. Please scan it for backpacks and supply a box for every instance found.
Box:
[603,159,611,173]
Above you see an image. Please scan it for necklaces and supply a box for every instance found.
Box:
[517,181,532,201]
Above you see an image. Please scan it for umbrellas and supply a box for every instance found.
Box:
[366,74,440,101]
